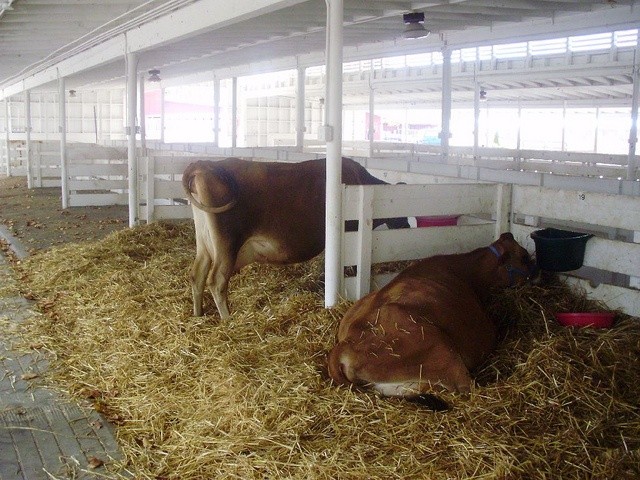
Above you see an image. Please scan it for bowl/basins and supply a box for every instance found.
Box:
[554,310,615,330]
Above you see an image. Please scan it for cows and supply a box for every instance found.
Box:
[328,231,537,412]
[181,157,410,322]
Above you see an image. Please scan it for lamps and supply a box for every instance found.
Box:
[68,89,76,98]
[402,12,430,40]
[148,70,161,82]
[480,91,487,101]
